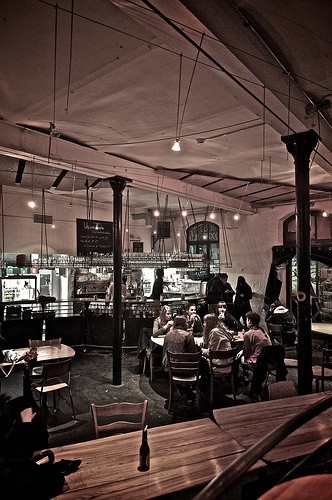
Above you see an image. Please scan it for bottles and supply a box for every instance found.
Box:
[194,315,201,322]
[30,345,37,362]
[139,428,150,470]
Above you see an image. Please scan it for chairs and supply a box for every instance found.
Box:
[241,343,285,398]
[208,344,239,410]
[27,337,63,347]
[32,357,76,421]
[166,349,205,415]
[266,322,298,358]
[90,398,149,443]
[138,326,165,382]
[312,348,332,392]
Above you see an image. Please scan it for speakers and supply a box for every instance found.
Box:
[157,221,170,239]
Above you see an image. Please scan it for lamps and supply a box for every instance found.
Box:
[153,169,166,217]
[234,181,250,220]
[171,30,205,153]
[181,174,196,217]
[210,178,225,220]
[26,156,37,209]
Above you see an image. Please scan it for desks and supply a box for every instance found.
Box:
[1,344,76,397]
[311,322,332,362]
[211,389,332,475]
[35,400,268,500]
[150,334,246,349]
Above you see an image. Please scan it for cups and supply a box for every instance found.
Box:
[238,332,243,337]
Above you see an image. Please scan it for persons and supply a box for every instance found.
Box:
[183,303,205,337]
[216,301,242,334]
[163,316,201,374]
[234,312,271,386]
[149,269,165,299]
[200,313,232,374]
[152,305,174,337]
[107,274,128,301]
[265,301,296,344]
[206,272,235,315]
[233,276,252,326]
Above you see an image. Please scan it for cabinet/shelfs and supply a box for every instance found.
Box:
[162,279,201,295]
